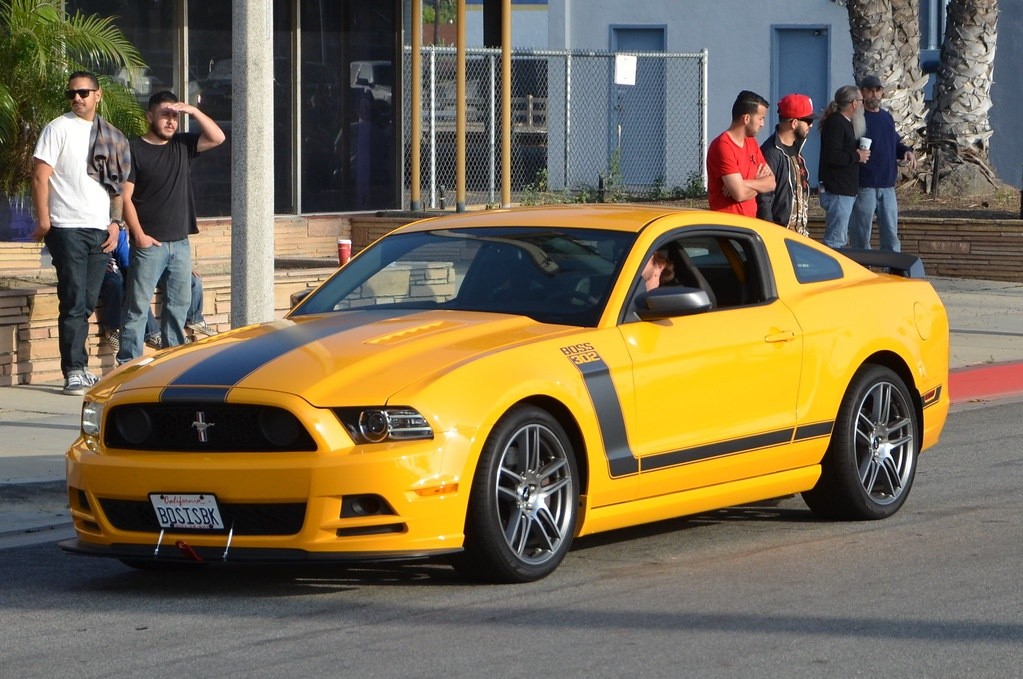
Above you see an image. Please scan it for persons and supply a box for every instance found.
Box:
[640,249,682,293]
[849,75,917,276]
[301,90,394,212]
[756,93,827,237]
[30,70,131,396]
[815,86,871,249]
[96,229,219,350]
[706,90,777,218]
[115,91,226,366]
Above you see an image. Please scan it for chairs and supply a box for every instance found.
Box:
[668,240,717,310]
[457,243,527,311]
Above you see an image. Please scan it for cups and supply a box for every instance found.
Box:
[337,239,352,268]
[860,137,872,151]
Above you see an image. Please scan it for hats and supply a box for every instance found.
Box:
[859,75,884,89]
[777,94,826,119]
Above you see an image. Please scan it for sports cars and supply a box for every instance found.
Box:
[54,195,954,586]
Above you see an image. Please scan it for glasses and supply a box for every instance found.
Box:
[789,118,814,127]
[850,99,865,104]
[65,89,97,99]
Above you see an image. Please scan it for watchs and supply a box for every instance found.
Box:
[111,219,126,231]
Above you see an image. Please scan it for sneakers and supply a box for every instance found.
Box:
[107,331,120,351]
[146,337,162,350]
[63,374,84,396]
[185,337,191,344]
[187,320,218,336]
[82,371,100,387]
